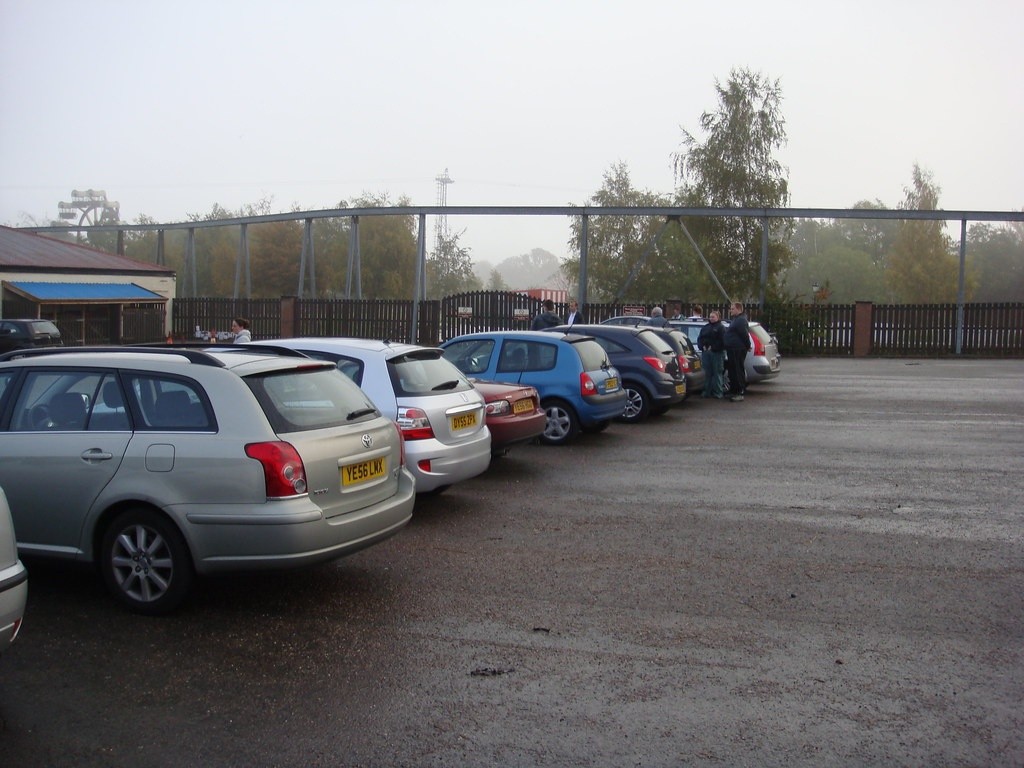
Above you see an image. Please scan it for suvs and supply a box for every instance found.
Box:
[0,319,64,354]
[0,342,417,613]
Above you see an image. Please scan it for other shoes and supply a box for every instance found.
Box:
[713,391,724,399]
[725,392,745,403]
[700,391,712,398]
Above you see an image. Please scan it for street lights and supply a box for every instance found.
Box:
[812,282,819,303]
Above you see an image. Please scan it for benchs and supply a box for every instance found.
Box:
[150,391,208,427]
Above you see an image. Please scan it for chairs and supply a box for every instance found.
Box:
[47,393,87,430]
[98,382,129,430]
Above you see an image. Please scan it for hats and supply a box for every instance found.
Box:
[673,304,682,313]
[541,300,554,310]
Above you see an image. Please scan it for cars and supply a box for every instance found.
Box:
[601,316,651,325]
[541,324,689,423]
[653,327,705,392]
[240,337,491,497]
[471,381,546,456]
[438,331,627,446]
[668,321,781,387]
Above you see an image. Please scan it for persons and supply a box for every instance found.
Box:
[529,299,564,330]
[647,307,672,327]
[232,318,251,344]
[671,305,686,319]
[724,302,751,401]
[688,304,703,318]
[564,300,584,325]
[697,311,726,399]
[208,330,218,342]
